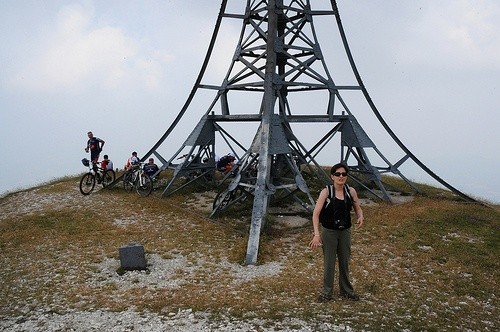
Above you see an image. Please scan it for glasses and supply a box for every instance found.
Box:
[333,172,347,177]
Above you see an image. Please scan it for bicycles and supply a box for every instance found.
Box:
[80,162,116,195]
[213,164,251,210]
[123,162,153,197]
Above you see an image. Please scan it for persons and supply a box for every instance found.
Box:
[203,153,237,175]
[310,163,364,302]
[84,132,160,183]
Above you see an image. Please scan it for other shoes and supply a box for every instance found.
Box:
[338,290,359,302]
[319,294,332,303]
